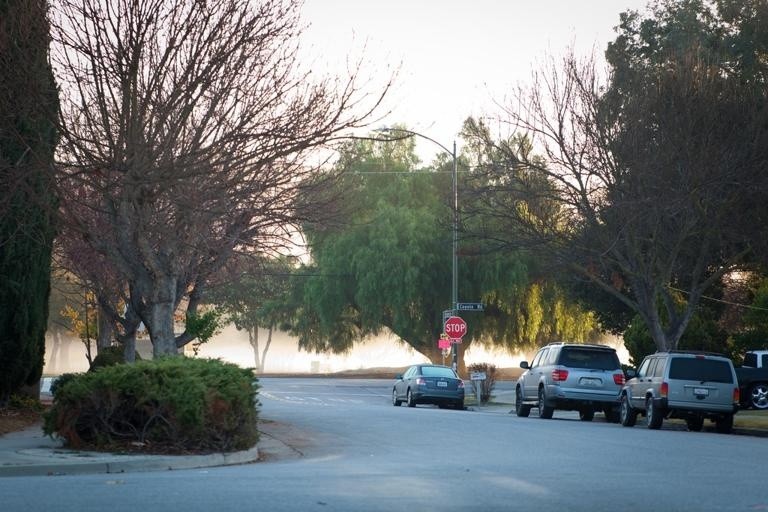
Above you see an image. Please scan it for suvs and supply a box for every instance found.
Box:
[618,348,740,435]
[514,342,625,419]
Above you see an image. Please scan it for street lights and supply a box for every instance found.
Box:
[372,127,459,369]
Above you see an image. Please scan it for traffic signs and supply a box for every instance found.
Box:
[456,302,485,312]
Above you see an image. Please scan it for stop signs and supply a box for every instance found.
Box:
[444,317,467,340]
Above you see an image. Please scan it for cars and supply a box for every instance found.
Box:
[741,349,767,369]
[392,364,465,408]
[733,367,767,410]
